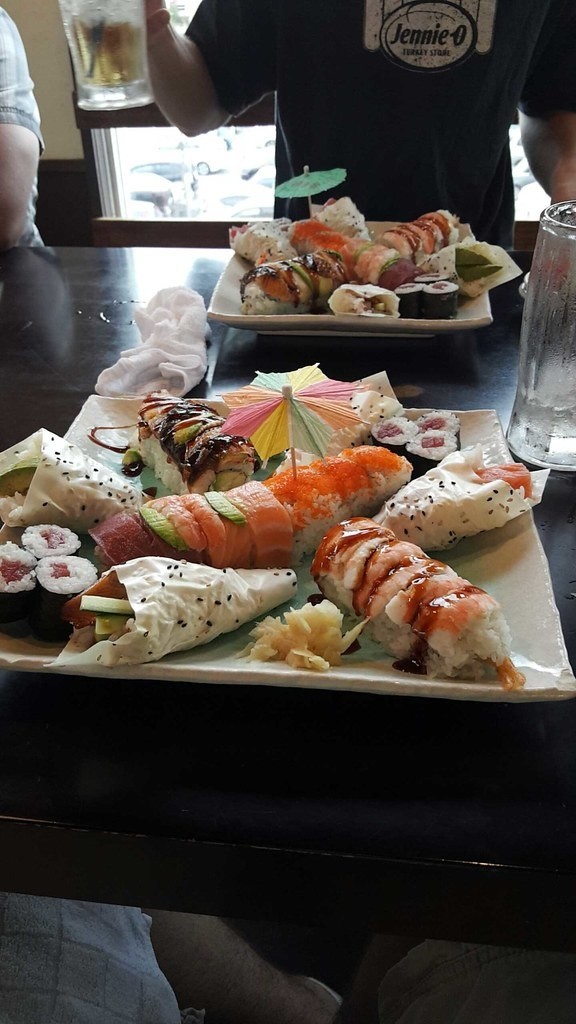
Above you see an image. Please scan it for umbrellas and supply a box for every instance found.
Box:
[276,164,347,219]
[216,361,370,479]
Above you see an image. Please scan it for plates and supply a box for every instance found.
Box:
[206,221,494,338]
[0,392,574,703]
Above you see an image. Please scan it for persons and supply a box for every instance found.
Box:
[145,0,575,254]
[0,4,48,249]
[0,887,576,1023]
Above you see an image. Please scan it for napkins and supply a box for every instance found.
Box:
[92,284,213,398]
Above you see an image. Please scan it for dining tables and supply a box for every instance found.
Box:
[0,244,575,959]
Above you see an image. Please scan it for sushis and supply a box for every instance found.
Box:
[228,197,523,320]
[0,392,520,691]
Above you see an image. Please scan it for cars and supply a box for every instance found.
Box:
[127,122,275,221]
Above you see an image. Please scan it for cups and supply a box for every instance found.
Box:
[505,199,576,472]
[58,0,154,111]
